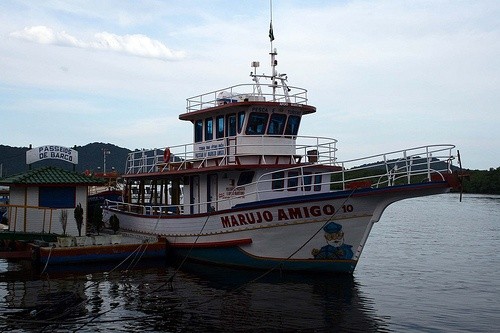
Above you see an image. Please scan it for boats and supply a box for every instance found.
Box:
[82,147,122,225]
[0,144,167,279]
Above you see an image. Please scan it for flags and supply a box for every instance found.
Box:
[269,19,275,41]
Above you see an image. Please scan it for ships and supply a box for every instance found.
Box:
[101,0,471,278]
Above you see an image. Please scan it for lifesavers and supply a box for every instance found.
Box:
[163,148,171,163]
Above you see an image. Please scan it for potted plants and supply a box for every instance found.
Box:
[93,204,107,245]
[57,210,72,248]
[74,203,88,247]
[109,214,122,245]
